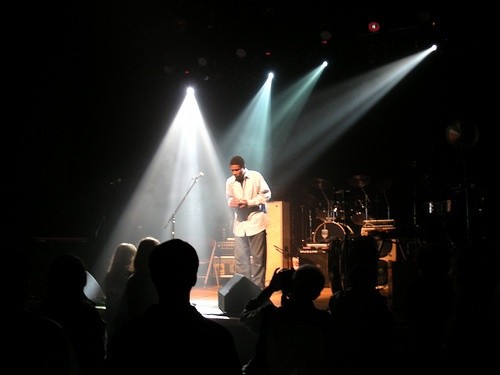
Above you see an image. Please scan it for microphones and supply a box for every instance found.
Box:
[192,172,204,180]
[110,179,121,184]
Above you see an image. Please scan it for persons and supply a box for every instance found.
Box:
[226,156,271,291]
[1,237,500,375]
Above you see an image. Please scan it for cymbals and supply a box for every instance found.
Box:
[348,176,370,188]
[309,177,327,189]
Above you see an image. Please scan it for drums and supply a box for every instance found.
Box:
[316,199,351,222]
[313,222,353,244]
[350,212,370,235]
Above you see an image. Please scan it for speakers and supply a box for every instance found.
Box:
[298,253,330,288]
[218,272,276,316]
[83,270,107,300]
